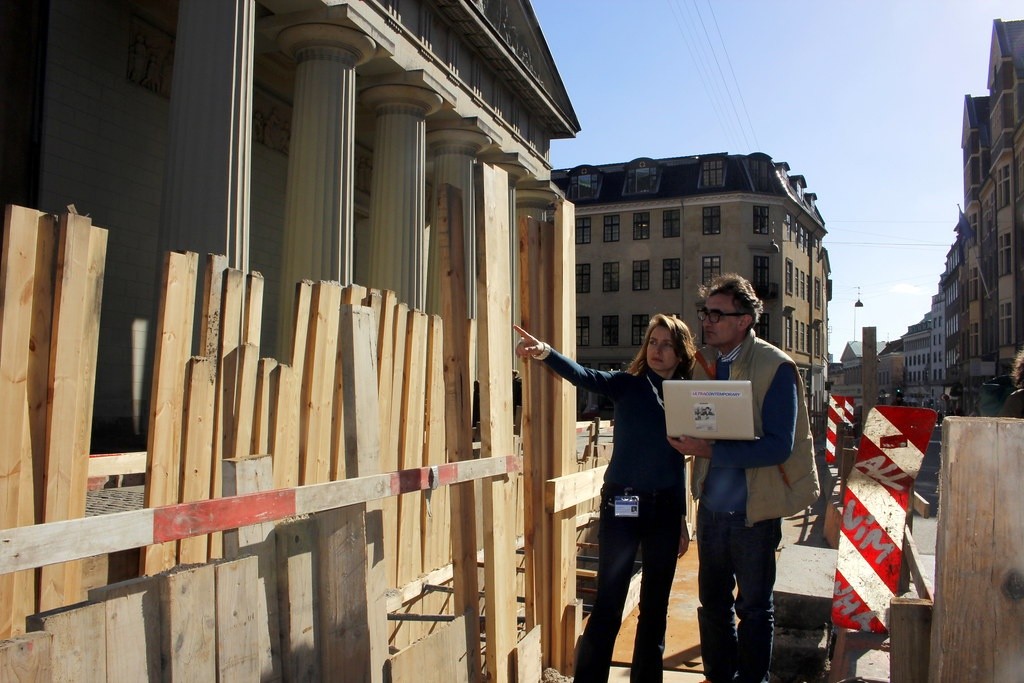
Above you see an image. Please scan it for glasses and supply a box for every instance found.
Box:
[697,310,748,323]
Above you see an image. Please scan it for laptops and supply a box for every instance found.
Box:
[662,380,761,441]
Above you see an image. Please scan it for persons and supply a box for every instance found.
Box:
[508,316,695,683]
[891,392,908,407]
[994,346,1024,419]
[666,269,818,681]
